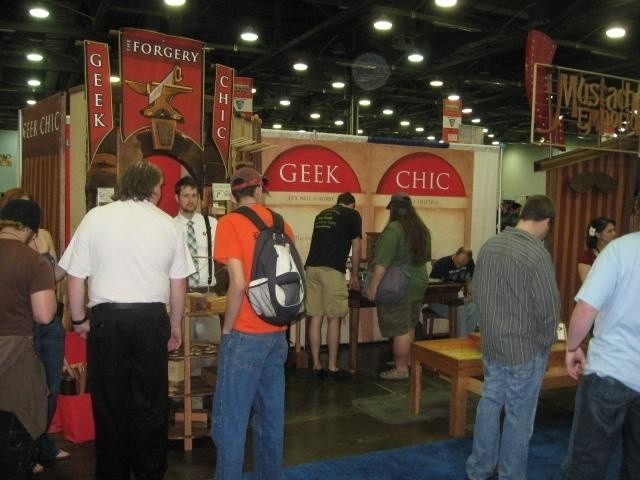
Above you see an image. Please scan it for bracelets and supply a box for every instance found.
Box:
[71,313,86,326]
[566,348,581,353]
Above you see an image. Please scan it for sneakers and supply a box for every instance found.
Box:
[328,366,349,377]
[313,366,327,379]
[382,368,409,380]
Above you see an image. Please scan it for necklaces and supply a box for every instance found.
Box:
[0,232,23,242]
[594,245,601,254]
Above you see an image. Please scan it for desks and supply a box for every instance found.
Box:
[409,333,589,438]
[306,280,466,374]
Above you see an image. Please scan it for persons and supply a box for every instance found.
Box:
[0,199,61,480]
[366,191,434,379]
[464,194,562,479]
[428,245,476,336]
[56,157,198,480]
[500,203,522,233]
[557,170,640,480]
[206,164,296,479]
[576,216,618,338]
[0,186,74,476]
[304,188,365,383]
[163,177,224,417]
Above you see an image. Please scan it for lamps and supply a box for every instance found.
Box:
[233,14,500,145]
[24,0,50,106]
[576,18,626,47]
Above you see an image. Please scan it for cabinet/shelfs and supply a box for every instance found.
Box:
[170,289,227,451]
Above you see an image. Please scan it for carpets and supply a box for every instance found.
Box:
[211,421,623,478]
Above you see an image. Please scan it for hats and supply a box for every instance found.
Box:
[1,199,42,232]
[386,192,413,209]
[230,167,265,192]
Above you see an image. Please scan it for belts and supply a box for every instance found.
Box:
[90,302,166,314]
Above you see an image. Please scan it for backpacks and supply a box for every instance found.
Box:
[230,207,306,326]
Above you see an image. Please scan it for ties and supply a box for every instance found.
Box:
[187,221,201,287]
